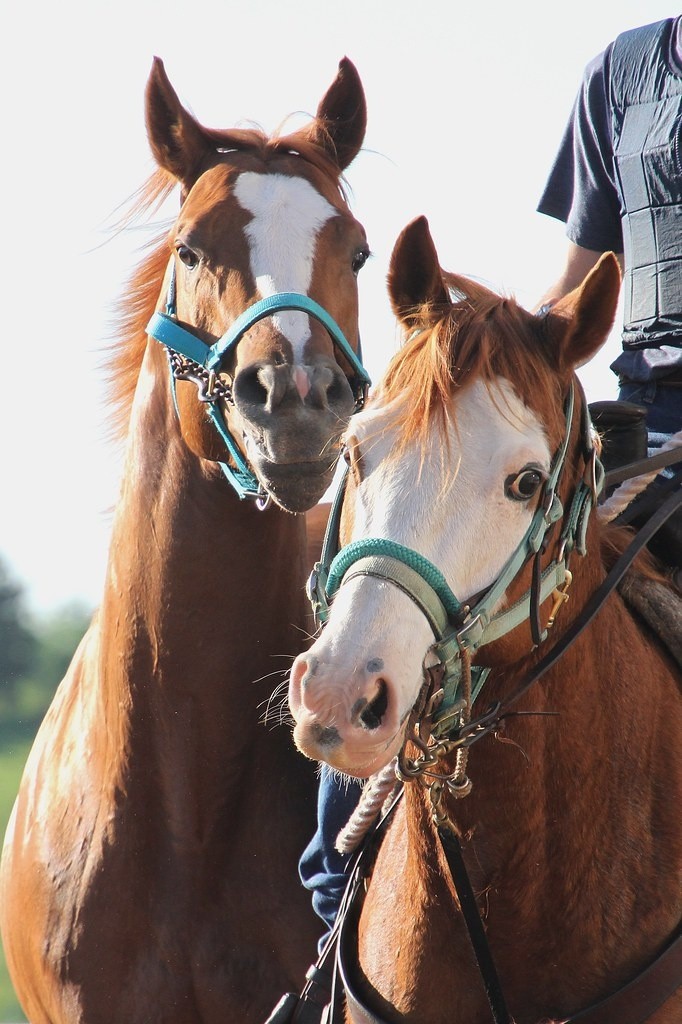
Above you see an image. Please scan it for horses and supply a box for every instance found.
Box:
[0,54,371,1024]
[278,214,682,1024]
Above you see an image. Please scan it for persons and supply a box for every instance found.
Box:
[300,13,682,958]
